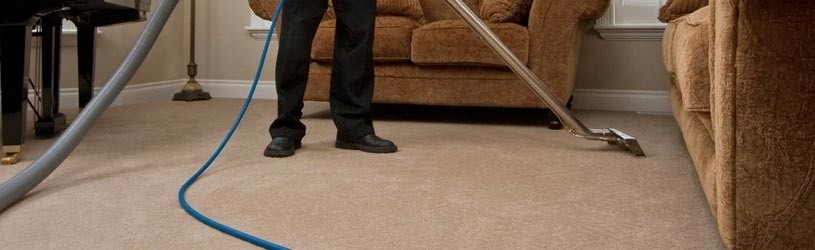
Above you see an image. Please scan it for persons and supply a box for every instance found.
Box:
[264,0,398,157]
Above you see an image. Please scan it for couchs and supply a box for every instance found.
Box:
[658,0,815,250]
[249,0,611,130]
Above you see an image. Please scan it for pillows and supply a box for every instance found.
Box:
[480,0,530,23]
[329,0,425,23]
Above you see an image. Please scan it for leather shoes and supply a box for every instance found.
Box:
[263,136,301,157]
[335,131,397,153]
